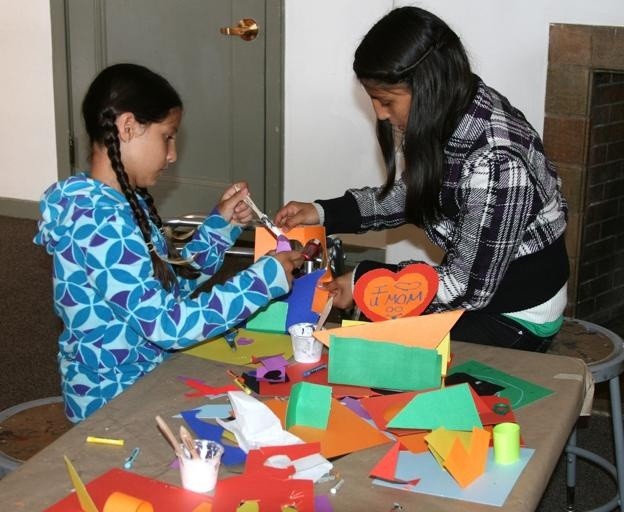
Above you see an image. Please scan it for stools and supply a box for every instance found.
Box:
[0,396,73,472]
[545,318,624,512]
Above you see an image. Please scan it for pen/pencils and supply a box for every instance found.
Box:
[304,363,325,377]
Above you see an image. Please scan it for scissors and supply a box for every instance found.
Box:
[222,326,238,351]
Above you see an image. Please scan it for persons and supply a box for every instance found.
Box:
[28,61,305,426]
[271,5,571,353]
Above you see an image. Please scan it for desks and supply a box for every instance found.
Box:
[0,322,588,512]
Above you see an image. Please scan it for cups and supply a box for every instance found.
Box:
[174,438,225,493]
[493,421,520,465]
[288,321,326,363]
